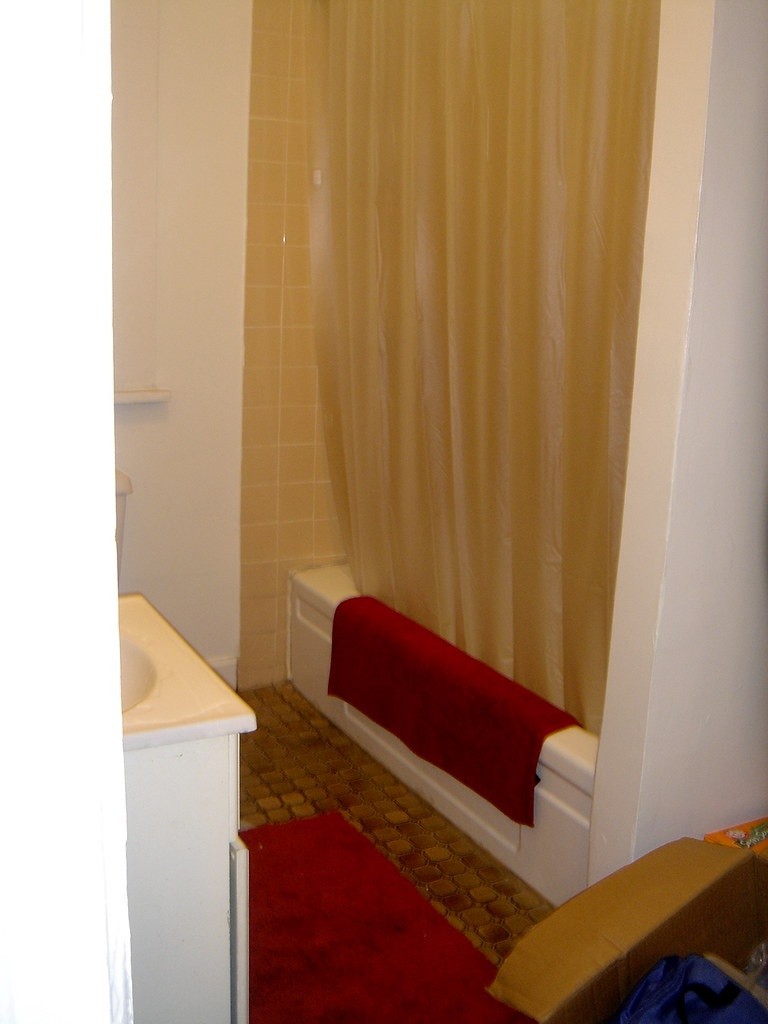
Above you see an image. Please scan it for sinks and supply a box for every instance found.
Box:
[119,592,164,719]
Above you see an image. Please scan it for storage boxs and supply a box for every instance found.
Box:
[486,816,768,1024]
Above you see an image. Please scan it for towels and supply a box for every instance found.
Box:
[328,595,589,830]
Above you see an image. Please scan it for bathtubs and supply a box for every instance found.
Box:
[288,565,602,909]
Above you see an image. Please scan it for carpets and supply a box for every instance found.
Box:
[240,812,536,1023]
